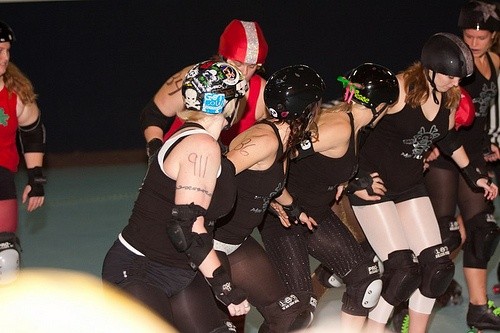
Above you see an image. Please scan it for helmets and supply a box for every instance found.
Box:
[219,19,268,65]
[458,0,500,30]
[264,65,326,118]
[0,22,13,42]
[182,60,249,114]
[422,32,474,78]
[337,63,399,109]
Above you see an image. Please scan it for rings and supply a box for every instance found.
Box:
[245,304,250,308]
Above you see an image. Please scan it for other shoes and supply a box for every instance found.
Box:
[390,299,408,333]
[467,299,500,331]
[438,280,463,306]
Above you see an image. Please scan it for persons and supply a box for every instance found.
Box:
[140,20,269,157]
[0,21,45,277]
[313,0,499,333]
[258,63,399,333]
[101,61,251,333]
[216,65,325,333]
